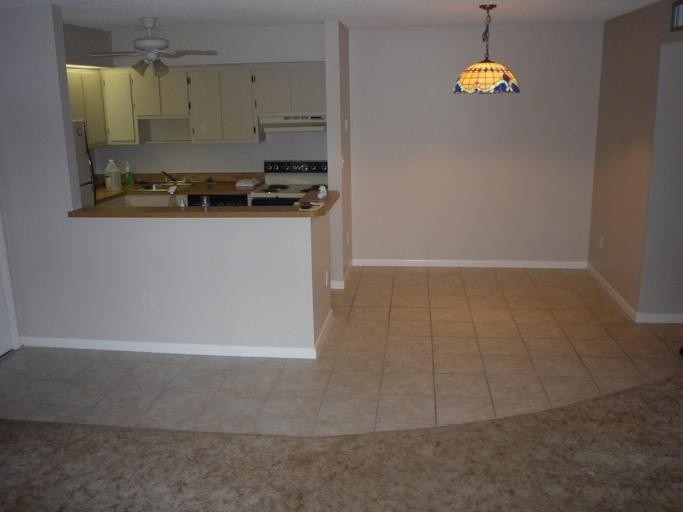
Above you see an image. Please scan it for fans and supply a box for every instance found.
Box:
[89,18,217,78]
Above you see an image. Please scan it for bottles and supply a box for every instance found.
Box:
[168,191,177,207]
[124,166,132,185]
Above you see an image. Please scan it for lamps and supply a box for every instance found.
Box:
[451,4,520,95]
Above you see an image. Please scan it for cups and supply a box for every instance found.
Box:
[200,194,210,208]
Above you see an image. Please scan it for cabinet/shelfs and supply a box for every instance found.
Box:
[67,68,106,149]
[97,194,126,208]
[126,194,188,208]
[133,66,189,119]
[189,63,259,145]
[255,63,326,123]
[103,68,140,145]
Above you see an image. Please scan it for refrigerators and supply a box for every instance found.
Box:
[71,118,97,208]
